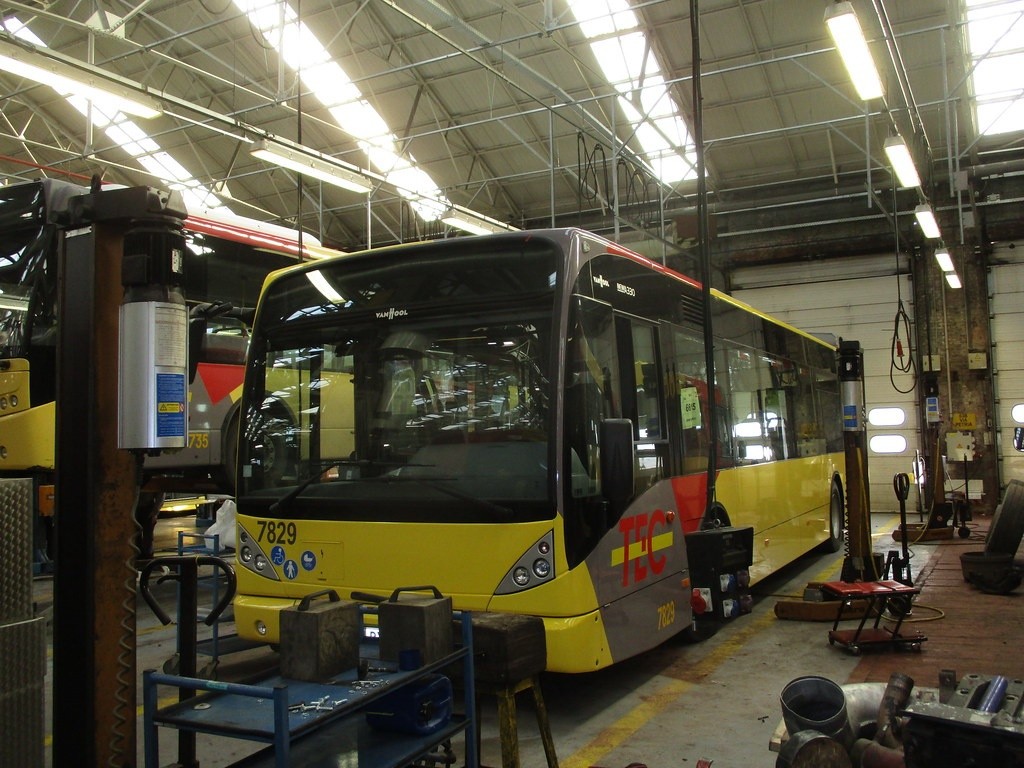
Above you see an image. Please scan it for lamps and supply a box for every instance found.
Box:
[881,132,921,189]
[935,246,964,289]
[248,138,373,193]
[824,2,886,99]
[914,204,943,243]
[1,38,164,120]
[443,204,513,237]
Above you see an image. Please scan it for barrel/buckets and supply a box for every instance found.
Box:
[364,672,454,737]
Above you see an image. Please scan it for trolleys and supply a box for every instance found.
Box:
[142,609,478,768]
[162,531,239,680]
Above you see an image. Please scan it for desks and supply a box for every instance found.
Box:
[819,579,929,655]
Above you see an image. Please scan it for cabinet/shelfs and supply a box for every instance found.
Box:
[140,607,480,768]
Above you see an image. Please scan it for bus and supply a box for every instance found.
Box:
[0,176,520,496]
[188,226,840,673]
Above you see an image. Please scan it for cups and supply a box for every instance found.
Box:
[399,649,420,671]
